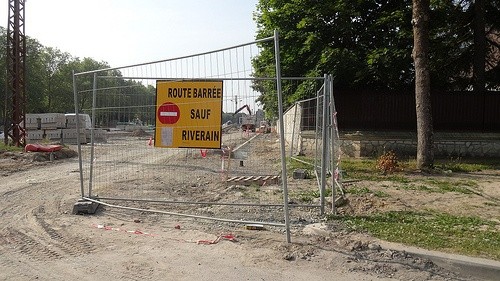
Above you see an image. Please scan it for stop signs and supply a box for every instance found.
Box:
[157,100,181,125]
